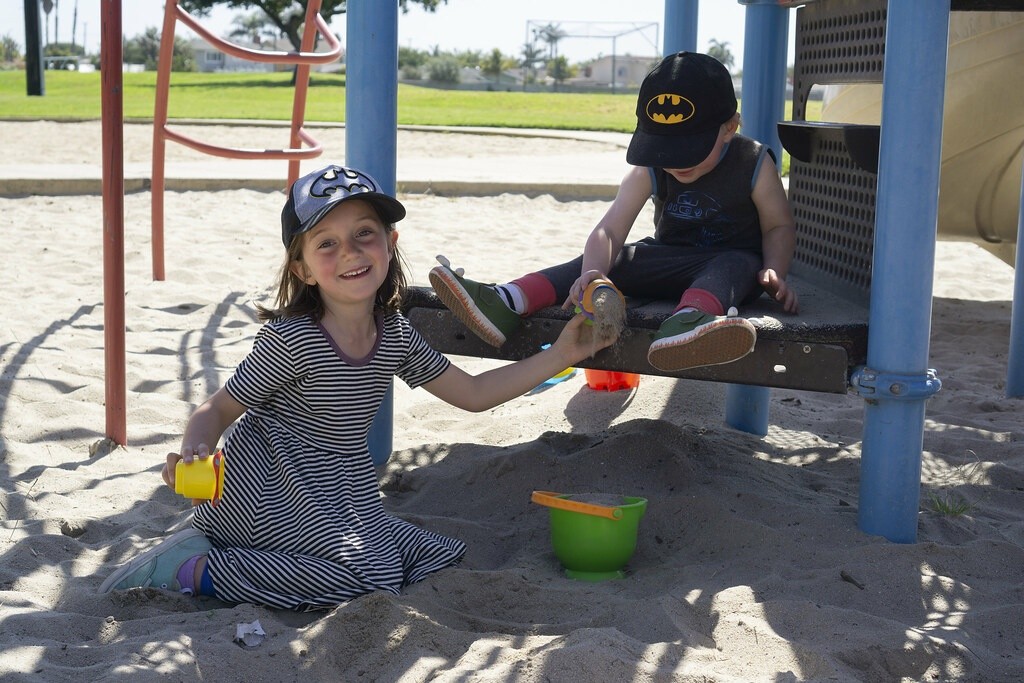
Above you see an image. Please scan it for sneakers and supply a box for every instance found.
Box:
[98,529,213,596]
[648,306,757,372]
[428,255,523,349]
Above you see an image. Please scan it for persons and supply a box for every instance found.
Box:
[96,165,623,611]
[429,51,797,374]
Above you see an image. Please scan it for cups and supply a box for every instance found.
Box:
[174,453,224,507]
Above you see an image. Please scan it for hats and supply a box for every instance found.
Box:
[281,164,407,249]
[626,52,738,169]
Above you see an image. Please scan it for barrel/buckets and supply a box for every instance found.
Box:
[530,490,648,582]
